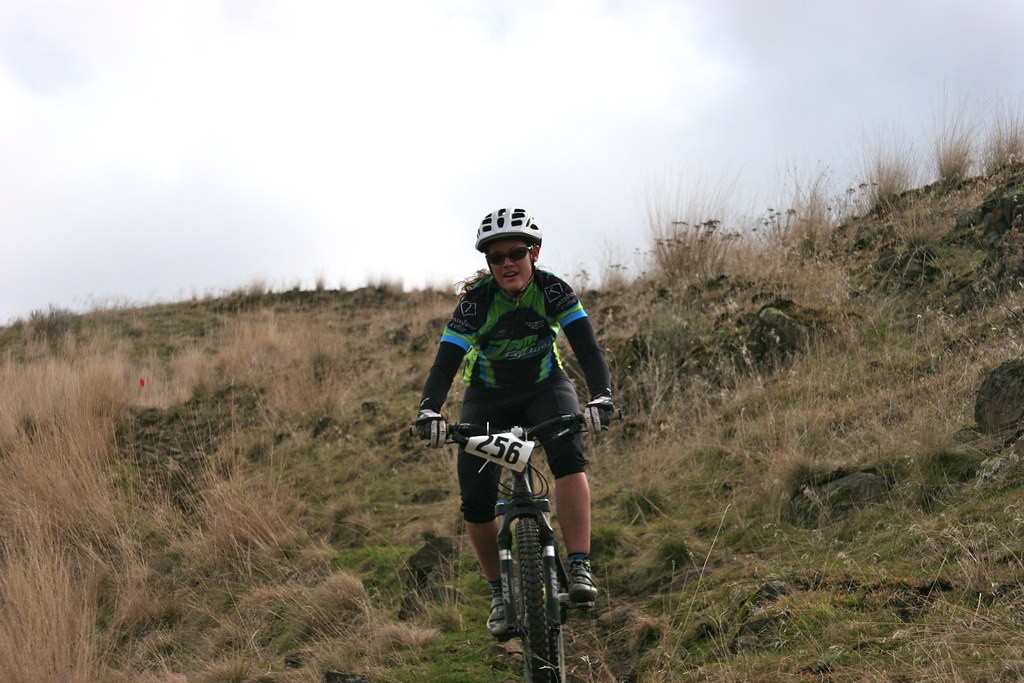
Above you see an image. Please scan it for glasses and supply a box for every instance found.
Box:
[485,245,534,264]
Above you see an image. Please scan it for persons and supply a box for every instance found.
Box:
[415,209,614,637]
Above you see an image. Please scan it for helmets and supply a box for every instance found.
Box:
[474,207,542,253]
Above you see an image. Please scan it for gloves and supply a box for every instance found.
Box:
[584,387,614,435]
[415,409,449,450]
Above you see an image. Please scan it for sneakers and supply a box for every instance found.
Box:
[568,568,597,613]
[487,597,511,639]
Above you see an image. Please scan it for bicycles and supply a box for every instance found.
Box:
[408,407,623,683]
